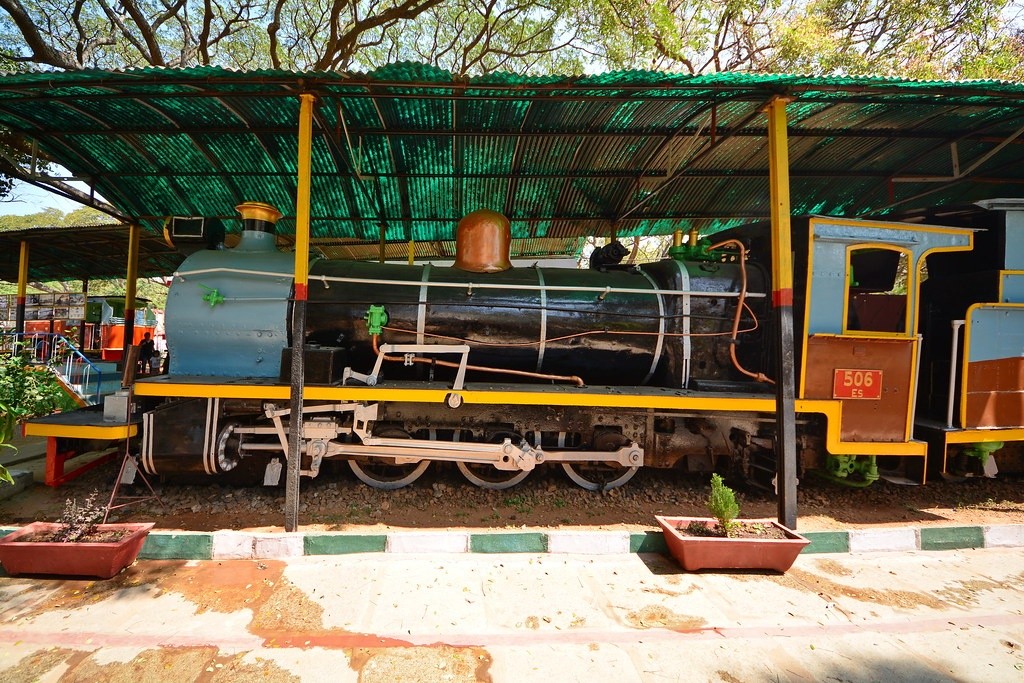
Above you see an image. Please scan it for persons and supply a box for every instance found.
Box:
[138,332,170,377]
[34,334,49,347]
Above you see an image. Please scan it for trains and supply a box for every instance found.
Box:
[128,192,1024,493]
